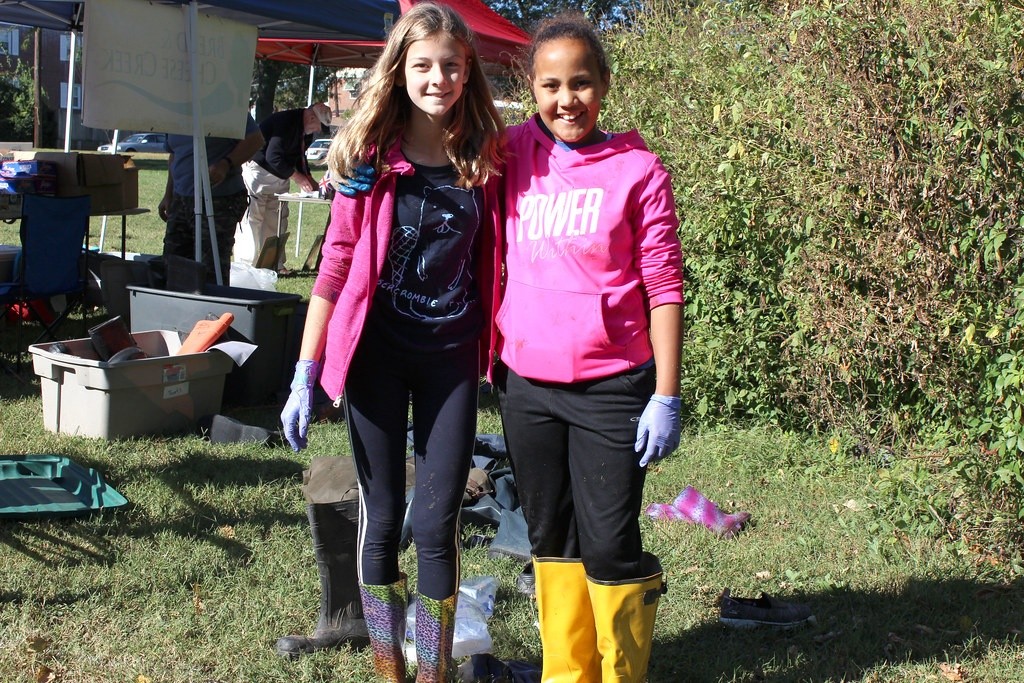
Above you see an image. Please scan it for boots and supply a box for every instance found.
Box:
[586,551,667,683]
[392,423,532,565]
[50,306,343,450]
[531,552,600,683]
[274,497,371,657]
[415,589,457,683]
[645,503,695,526]
[359,572,408,683]
[673,483,752,540]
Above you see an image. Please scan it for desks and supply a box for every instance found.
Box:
[81,208,150,294]
[275,197,333,269]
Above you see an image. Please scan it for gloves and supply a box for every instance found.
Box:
[326,161,375,195]
[634,394,681,467]
[281,360,319,455]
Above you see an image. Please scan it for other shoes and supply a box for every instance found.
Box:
[719,587,812,628]
[276,266,299,278]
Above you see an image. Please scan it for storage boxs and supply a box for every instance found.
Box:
[121,283,305,406]
[0,141,140,219]
[29,328,233,441]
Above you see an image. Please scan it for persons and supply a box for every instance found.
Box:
[332,19,684,683]
[233,102,332,276]
[158,112,264,286]
[281,4,504,683]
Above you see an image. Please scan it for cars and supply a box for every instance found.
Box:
[96,133,167,152]
[306,138,333,161]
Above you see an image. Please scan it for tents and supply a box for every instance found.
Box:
[0,0,538,286]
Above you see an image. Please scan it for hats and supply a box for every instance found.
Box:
[310,101,333,136]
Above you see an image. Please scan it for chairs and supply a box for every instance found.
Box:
[0,193,98,387]
